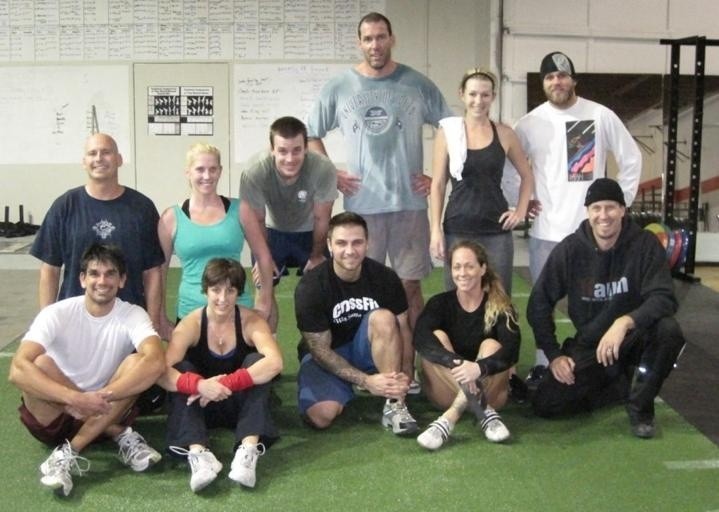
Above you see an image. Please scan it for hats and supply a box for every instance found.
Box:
[540,52,575,79]
[584,178,626,206]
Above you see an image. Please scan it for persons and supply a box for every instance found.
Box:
[497,50,643,397]
[427,67,535,403]
[155,256,285,494]
[239,116,339,343]
[526,178,684,441]
[27,132,177,338]
[306,13,453,395]
[6,239,166,497]
[291,209,423,436]
[155,142,276,326]
[408,238,524,452]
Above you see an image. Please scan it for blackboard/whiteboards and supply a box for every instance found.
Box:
[231,63,370,215]
[0,65,135,163]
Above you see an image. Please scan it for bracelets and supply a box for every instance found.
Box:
[217,368,255,393]
[175,372,205,394]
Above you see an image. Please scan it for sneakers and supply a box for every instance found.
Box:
[228,443,260,489]
[416,416,450,450]
[480,409,510,442]
[187,447,223,493]
[381,398,418,435]
[40,444,76,497]
[505,373,527,403]
[523,365,550,391]
[405,367,423,394]
[631,414,655,438]
[118,428,162,472]
